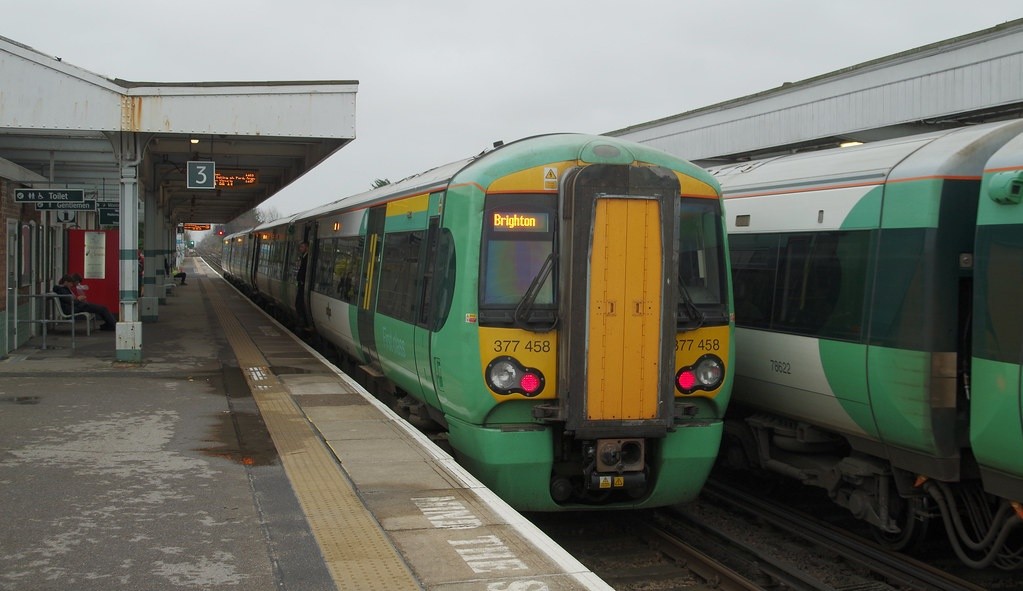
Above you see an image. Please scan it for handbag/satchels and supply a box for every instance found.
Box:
[76,285,87,302]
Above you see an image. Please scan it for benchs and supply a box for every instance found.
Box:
[165,274,181,297]
[47,293,97,336]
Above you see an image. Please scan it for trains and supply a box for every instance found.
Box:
[221,132,735,513]
[707,118,1022,568]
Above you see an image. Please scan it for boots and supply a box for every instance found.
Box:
[181,280,188,286]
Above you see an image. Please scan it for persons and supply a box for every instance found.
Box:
[171,266,188,285]
[339,270,360,304]
[71,273,88,303]
[52,275,117,328]
[294,242,309,316]
[138,256,144,297]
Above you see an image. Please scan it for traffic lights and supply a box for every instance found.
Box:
[219,231,223,235]
